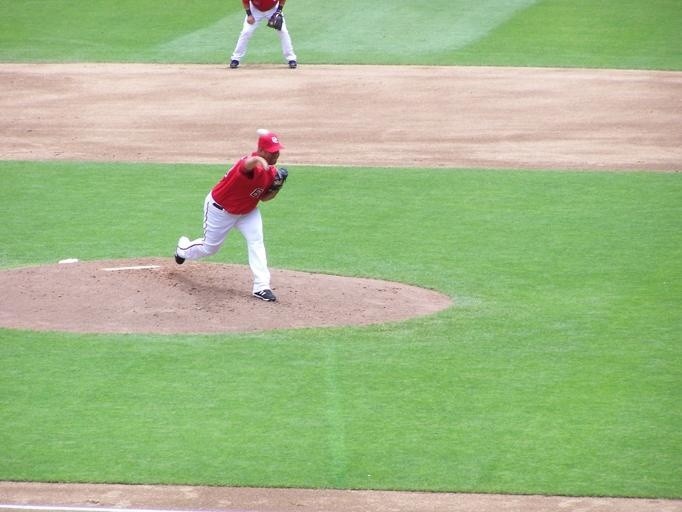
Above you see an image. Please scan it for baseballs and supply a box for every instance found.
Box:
[257,128,268,137]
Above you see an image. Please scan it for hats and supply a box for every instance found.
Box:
[258,132,284,153]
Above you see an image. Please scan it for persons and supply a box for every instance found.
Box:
[175,128,288,302]
[229,0,297,69]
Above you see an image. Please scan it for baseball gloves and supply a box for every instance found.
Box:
[269,167,288,192]
[266,11,282,30]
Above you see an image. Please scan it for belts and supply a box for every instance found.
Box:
[213,203,223,210]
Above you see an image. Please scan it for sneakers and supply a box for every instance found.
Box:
[252,290,276,301]
[289,61,296,68]
[230,60,238,68]
[175,236,190,264]
